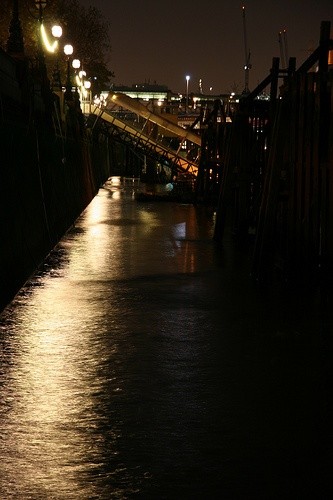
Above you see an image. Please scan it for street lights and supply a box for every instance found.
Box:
[35,0,46,64]
[185,76,190,114]
[51,24,61,91]
[73,58,91,115]
[64,43,73,94]
